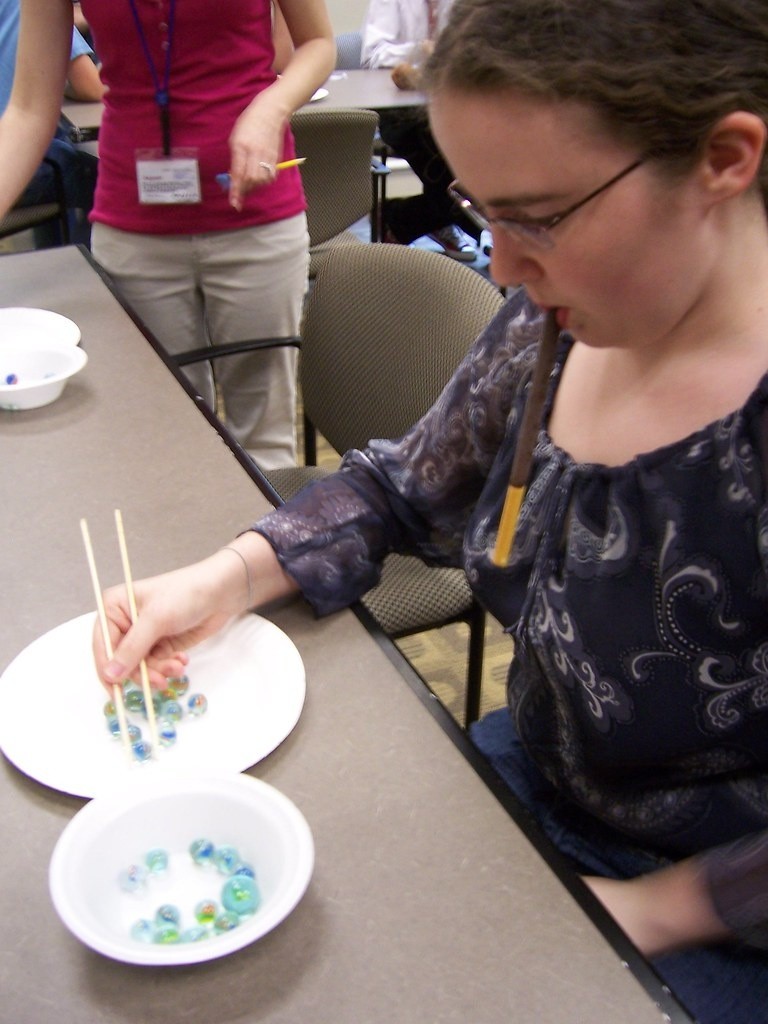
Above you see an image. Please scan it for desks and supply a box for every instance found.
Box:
[0,241,691,1024]
[58,65,426,240]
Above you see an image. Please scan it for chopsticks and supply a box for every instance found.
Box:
[80,509,161,762]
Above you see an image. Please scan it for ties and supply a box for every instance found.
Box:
[426,0,439,38]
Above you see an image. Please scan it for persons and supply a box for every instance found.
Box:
[1,0,336,471]
[96,0,768,1024]
[359,0,493,263]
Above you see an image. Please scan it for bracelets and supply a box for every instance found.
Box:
[218,545,252,613]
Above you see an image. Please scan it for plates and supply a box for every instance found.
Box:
[0,335,88,410]
[49,769,316,966]
[0,608,306,800]
[308,88,329,102]
[0,307,81,346]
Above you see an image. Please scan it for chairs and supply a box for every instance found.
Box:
[172,239,509,728]
[1,200,67,258]
[291,107,379,275]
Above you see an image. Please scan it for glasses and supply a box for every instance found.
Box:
[447,146,664,254]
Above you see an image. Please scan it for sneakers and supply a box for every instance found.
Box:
[426,223,476,260]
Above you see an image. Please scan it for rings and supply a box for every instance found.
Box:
[259,161,271,171]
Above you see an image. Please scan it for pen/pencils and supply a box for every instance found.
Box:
[215,157,307,184]
